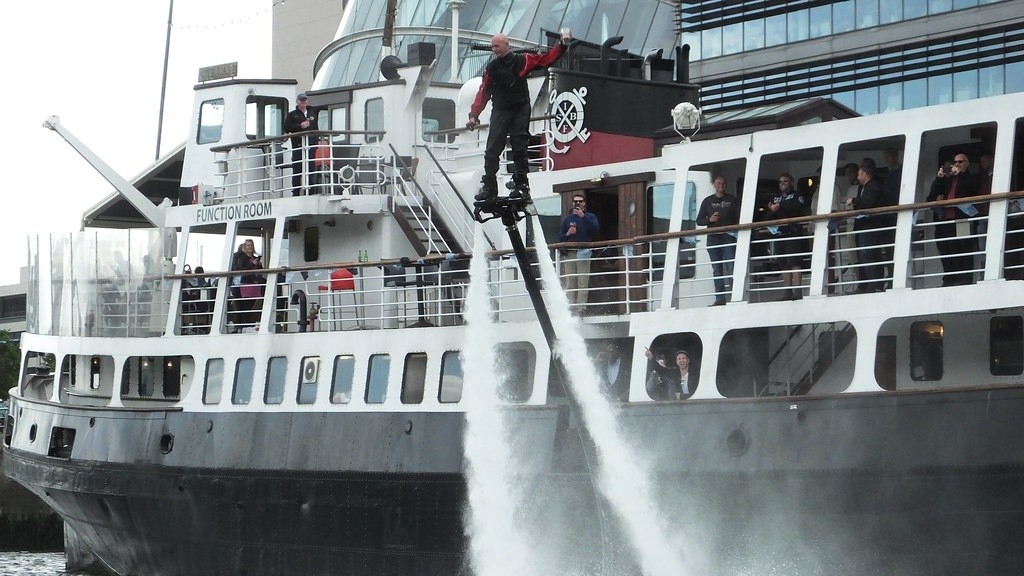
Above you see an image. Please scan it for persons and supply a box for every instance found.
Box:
[466,28,572,201]
[768,148,902,302]
[285,94,319,196]
[559,195,601,317]
[113,239,288,333]
[925,154,982,287]
[594,344,699,401]
[697,177,742,307]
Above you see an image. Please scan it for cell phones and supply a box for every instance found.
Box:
[575,203,580,210]
[257,255,262,261]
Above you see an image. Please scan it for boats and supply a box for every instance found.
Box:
[0,282,65,554]
[1,0,1024,576]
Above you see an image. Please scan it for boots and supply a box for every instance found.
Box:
[474,175,498,201]
[509,174,530,198]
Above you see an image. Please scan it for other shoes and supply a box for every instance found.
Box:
[776,288,803,300]
[708,296,726,307]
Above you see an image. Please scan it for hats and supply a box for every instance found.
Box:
[297,94,308,99]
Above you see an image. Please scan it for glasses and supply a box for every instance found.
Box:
[778,180,789,183]
[954,160,966,164]
[573,200,584,203]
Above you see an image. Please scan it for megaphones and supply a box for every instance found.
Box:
[381,55,407,80]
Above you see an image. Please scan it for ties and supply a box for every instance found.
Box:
[944,175,958,219]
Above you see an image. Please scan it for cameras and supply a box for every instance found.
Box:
[942,163,954,177]
